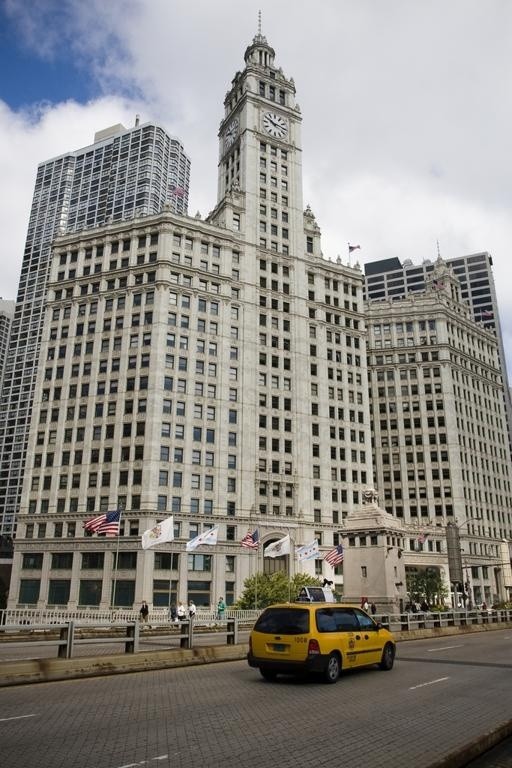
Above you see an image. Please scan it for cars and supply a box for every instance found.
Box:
[247,587,396,684]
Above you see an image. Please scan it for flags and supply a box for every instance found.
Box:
[142,516,174,549]
[81,509,120,534]
[185,525,218,551]
[324,543,344,567]
[296,540,319,562]
[349,245,360,253]
[263,535,290,557]
[240,530,259,550]
[418,532,427,544]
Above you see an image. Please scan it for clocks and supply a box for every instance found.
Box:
[262,111,289,140]
[222,118,240,150]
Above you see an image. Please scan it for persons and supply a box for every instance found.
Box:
[371,601,376,615]
[217,596,226,620]
[363,601,369,613]
[170,602,178,622]
[139,599,152,632]
[186,599,197,620]
[481,601,487,611]
[177,601,186,621]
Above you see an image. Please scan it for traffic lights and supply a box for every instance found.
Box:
[465,582,470,592]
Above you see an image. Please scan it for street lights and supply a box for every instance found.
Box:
[458,517,481,529]
